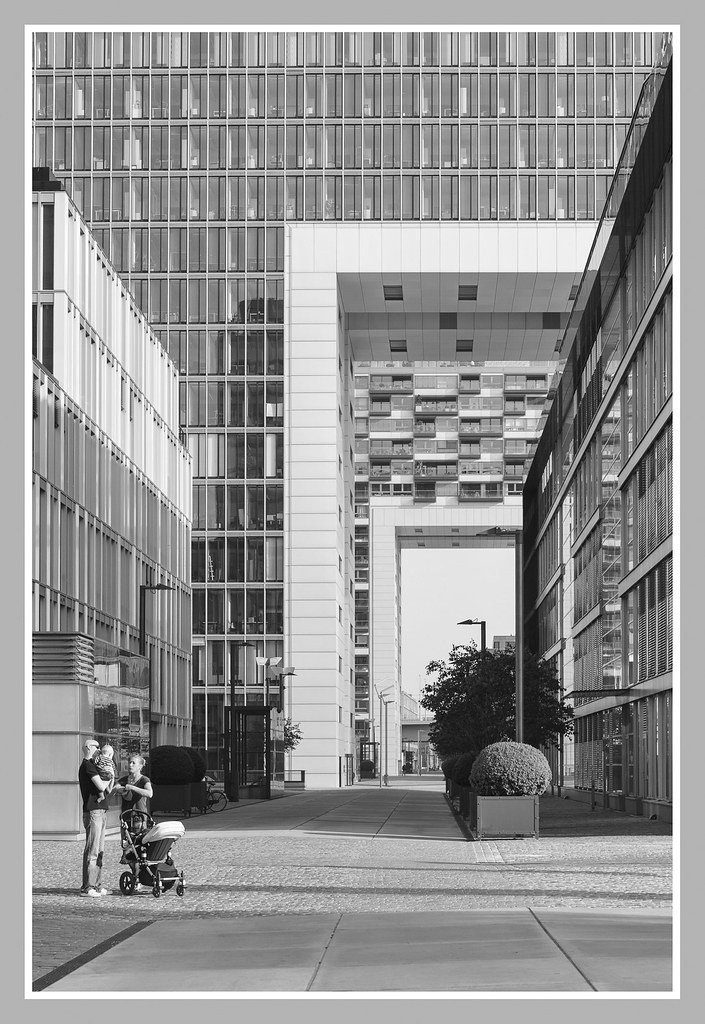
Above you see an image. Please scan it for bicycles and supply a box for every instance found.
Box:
[197,781,229,813]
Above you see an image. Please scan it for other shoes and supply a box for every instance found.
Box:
[100,889,108,895]
[80,889,101,898]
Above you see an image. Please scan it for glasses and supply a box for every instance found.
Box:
[86,745,100,749]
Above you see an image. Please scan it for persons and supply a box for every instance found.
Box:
[115,755,153,891]
[78,739,118,897]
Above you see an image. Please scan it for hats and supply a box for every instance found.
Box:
[121,787,132,801]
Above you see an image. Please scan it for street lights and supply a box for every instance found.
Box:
[279,672,298,709]
[230,642,255,802]
[417,729,425,777]
[379,693,390,788]
[457,619,486,653]
[139,582,177,656]
[385,700,395,786]
[264,677,279,799]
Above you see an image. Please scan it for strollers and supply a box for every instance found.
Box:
[119,808,188,898]
